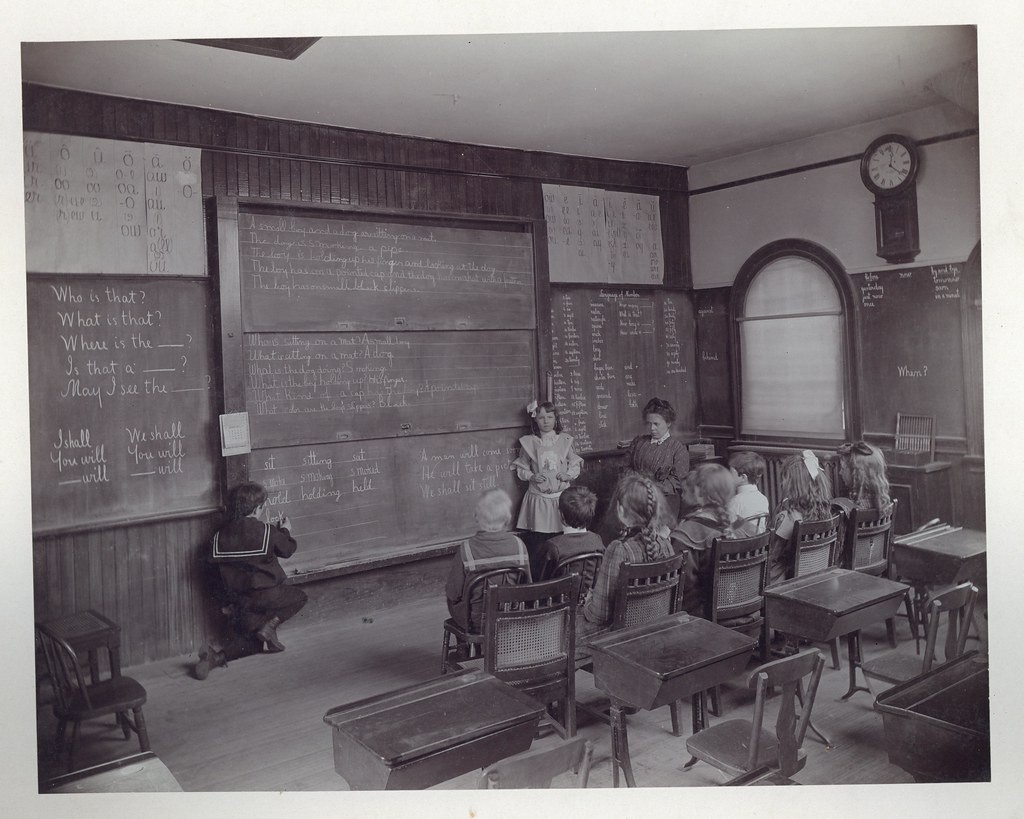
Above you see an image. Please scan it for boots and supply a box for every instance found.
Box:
[194,642,229,681]
[256,615,286,652]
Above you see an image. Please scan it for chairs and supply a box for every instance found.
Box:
[35,622,151,771]
[437,496,981,787]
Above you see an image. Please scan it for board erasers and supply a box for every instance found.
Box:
[617,439,632,449]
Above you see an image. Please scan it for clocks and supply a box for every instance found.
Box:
[859,132,922,266]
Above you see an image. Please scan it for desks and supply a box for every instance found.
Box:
[323,673,546,790]
[884,448,951,537]
[893,523,986,658]
[873,651,990,783]
[35,607,127,696]
[760,566,910,747]
[587,611,758,787]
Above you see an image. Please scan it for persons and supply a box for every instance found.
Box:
[447,487,535,667]
[618,397,690,522]
[195,482,308,681]
[534,443,895,649]
[510,399,585,570]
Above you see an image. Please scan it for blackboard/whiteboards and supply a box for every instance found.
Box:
[25,270,229,541]
[536,290,699,462]
[856,262,966,444]
[696,287,734,439]
[218,196,554,586]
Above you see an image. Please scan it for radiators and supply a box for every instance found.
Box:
[751,455,838,521]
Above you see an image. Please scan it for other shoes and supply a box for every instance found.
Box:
[449,645,470,662]
